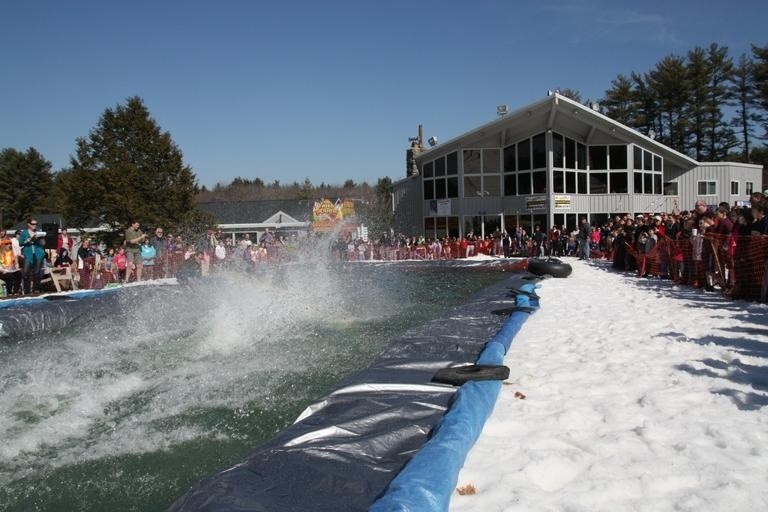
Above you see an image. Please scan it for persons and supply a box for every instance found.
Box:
[2,193,768,305]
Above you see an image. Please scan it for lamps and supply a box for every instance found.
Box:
[496,105,507,117]
[428,136,438,147]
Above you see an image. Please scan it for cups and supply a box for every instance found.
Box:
[692,229,697,236]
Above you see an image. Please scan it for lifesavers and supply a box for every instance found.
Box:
[431,365,510,386]
[521,258,572,280]
[511,289,540,300]
[491,307,535,315]
